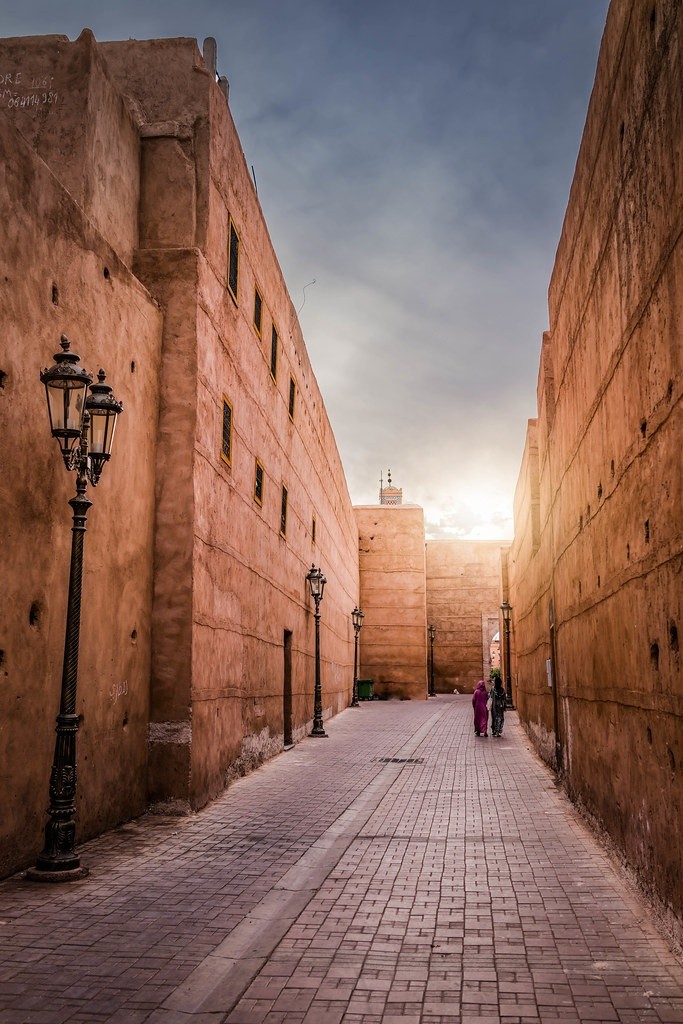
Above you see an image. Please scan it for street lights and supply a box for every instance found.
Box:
[24,332,127,887]
[306,562,330,738]
[499,599,516,711]
[350,605,365,707]
[427,624,437,697]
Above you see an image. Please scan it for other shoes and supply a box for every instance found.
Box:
[492,733,501,737]
[484,733,488,737]
[475,732,481,736]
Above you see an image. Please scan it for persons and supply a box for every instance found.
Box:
[472,681,488,737]
[488,677,507,737]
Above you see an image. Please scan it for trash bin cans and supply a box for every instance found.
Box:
[358,679,374,701]
